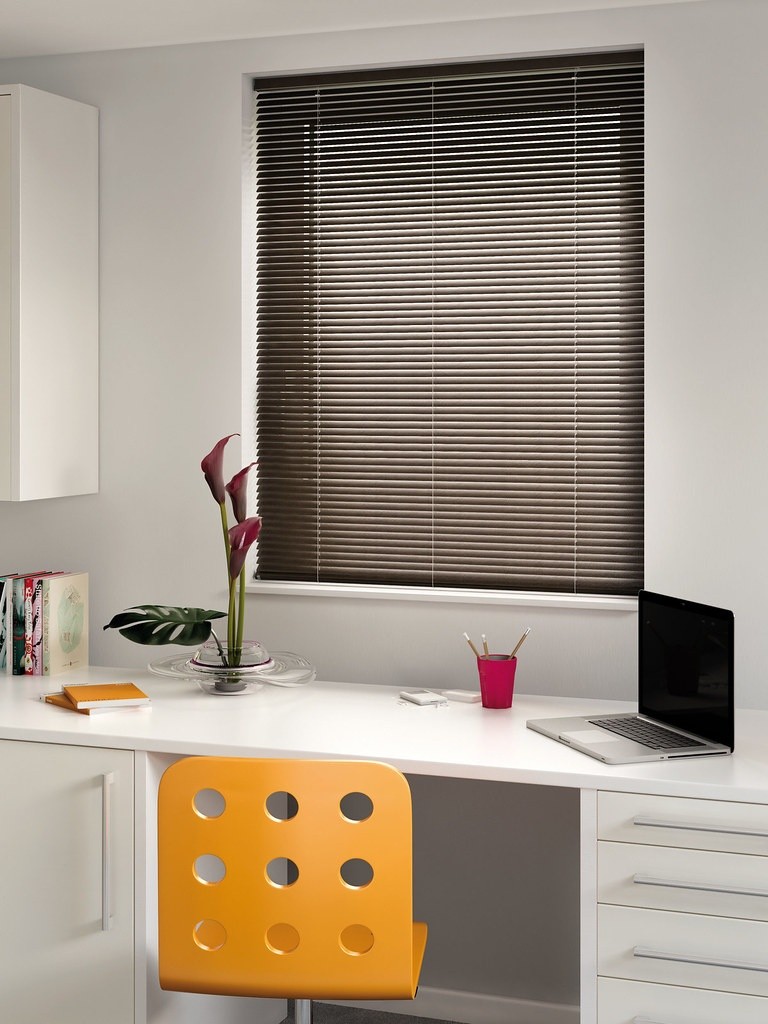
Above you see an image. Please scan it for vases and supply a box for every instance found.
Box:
[148,638,318,696]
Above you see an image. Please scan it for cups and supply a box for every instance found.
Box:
[477,654,517,708]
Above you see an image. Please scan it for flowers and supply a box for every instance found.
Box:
[102,433,263,682]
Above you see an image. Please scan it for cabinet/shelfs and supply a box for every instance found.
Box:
[0,84,99,502]
[0,738,289,1024]
[578,788,768,1024]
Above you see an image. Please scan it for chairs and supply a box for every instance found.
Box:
[158,756,427,1024]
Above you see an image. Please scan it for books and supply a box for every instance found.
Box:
[40,682,152,716]
[0,571,89,678]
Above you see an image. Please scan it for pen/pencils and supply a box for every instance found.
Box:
[507,627,531,660]
[463,633,482,659]
[481,634,490,660]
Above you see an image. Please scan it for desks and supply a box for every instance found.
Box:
[0,665,768,1024]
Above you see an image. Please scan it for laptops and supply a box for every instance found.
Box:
[525,589,735,765]
